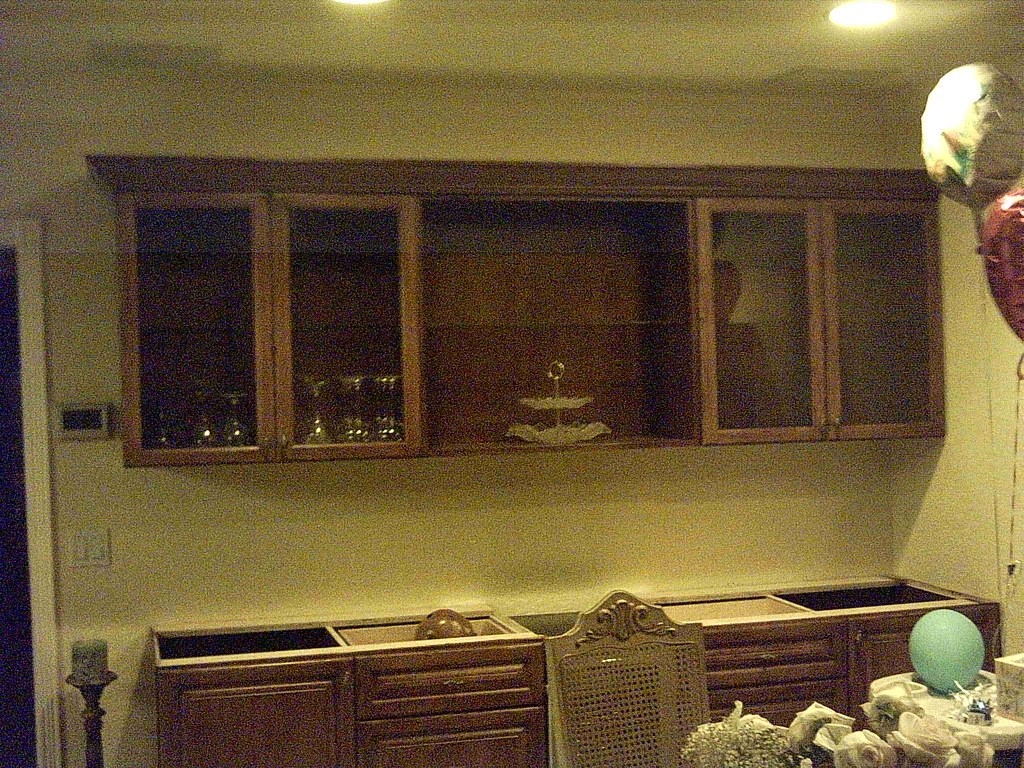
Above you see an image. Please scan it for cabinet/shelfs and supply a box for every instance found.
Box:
[82,147,950,470]
[152,574,1003,768]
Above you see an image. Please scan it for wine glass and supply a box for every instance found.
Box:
[161,392,249,446]
[295,369,398,442]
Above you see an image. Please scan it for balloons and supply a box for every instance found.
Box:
[909,609,985,693]
[920,61,1024,340]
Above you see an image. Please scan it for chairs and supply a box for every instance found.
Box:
[544,589,710,768]
